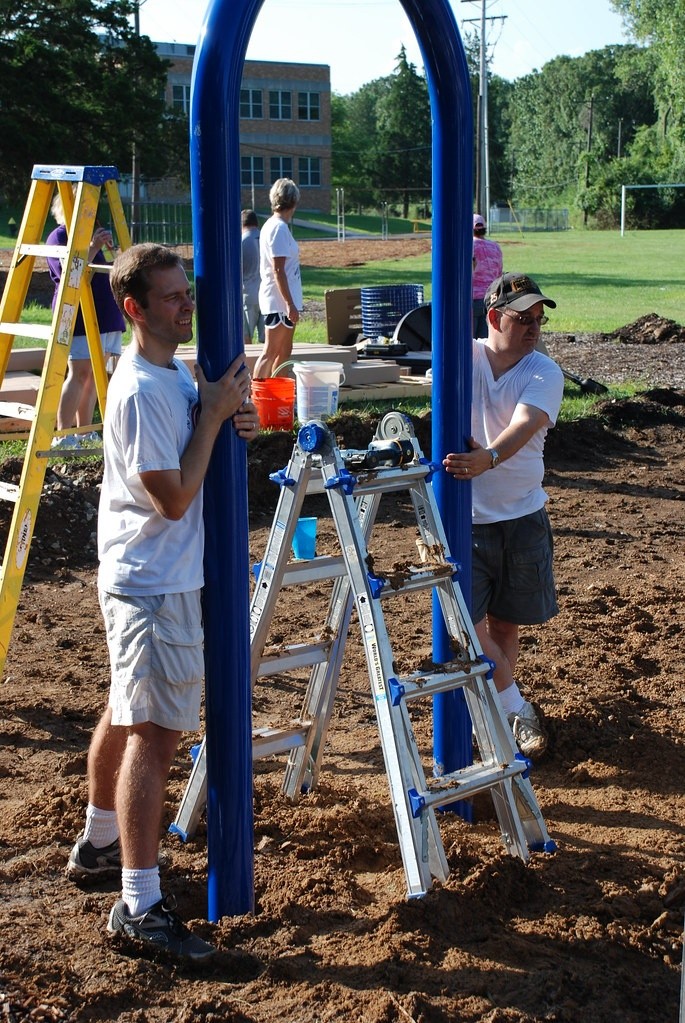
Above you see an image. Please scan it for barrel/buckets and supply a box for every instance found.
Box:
[292,361,346,425]
[250,378,296,431]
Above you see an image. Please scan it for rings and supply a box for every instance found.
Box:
[465,468,468,473]
[252,423,255,429]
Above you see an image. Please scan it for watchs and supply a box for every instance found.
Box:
[485,447,500,469]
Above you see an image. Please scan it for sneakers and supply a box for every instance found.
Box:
[106,892,215,970]
[508,701,546,757]
[65,829,170,886]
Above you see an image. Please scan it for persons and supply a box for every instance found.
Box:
[472,214,503,339]
[45,184,126,450]
[442,272,565,760]
[241,209,265,344]
[253,178,303,380]
[66,242,260,958]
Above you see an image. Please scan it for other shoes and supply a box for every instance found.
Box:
[50,435,84,450]
[74,431,102,444]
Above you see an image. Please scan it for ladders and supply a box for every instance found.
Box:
[168,410,558,895]
[1,161,138,671]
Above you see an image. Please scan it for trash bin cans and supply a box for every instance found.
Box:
[360,283,425,343]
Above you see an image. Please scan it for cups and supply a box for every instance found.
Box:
[291,517,318,559]
[100,230,116,263]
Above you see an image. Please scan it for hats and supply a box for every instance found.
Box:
[473,214,487,229]
[483,272,556,312]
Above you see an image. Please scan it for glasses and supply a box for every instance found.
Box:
[495,308,549,325]
